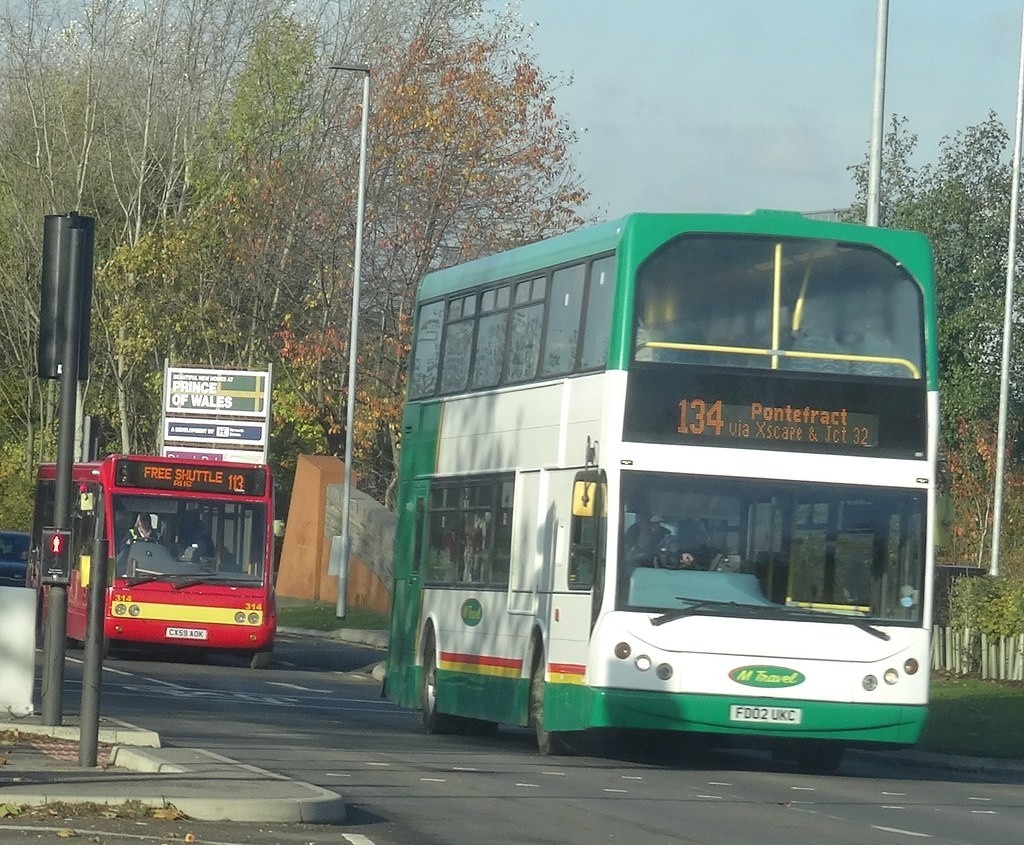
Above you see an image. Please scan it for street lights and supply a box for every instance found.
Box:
[329,60,371,619]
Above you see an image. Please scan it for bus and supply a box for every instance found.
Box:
[385,206,955,770]
[27,454,287,671]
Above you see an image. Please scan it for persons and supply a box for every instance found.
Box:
[118,514,169,554]
[172,499,215,562]
[627,516,711,570]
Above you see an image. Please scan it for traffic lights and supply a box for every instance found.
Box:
[42,525,74,586]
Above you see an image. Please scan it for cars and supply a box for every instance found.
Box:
[0,531,30,587]
